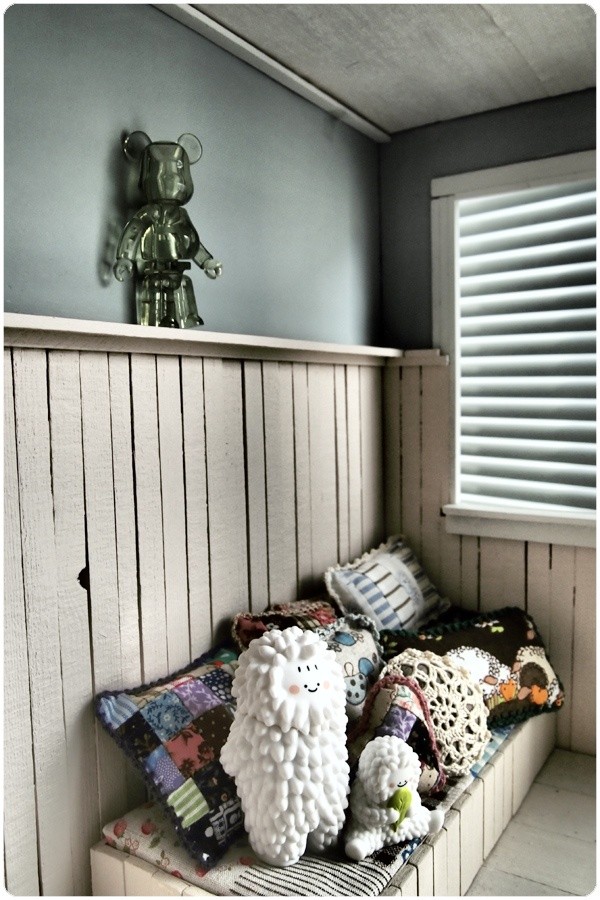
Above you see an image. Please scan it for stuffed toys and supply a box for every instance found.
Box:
[219,626,348,867]
[346,736,446,861]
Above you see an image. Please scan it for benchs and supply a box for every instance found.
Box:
[86,691,555,897]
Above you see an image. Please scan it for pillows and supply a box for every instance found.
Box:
[90,534,566,870]
